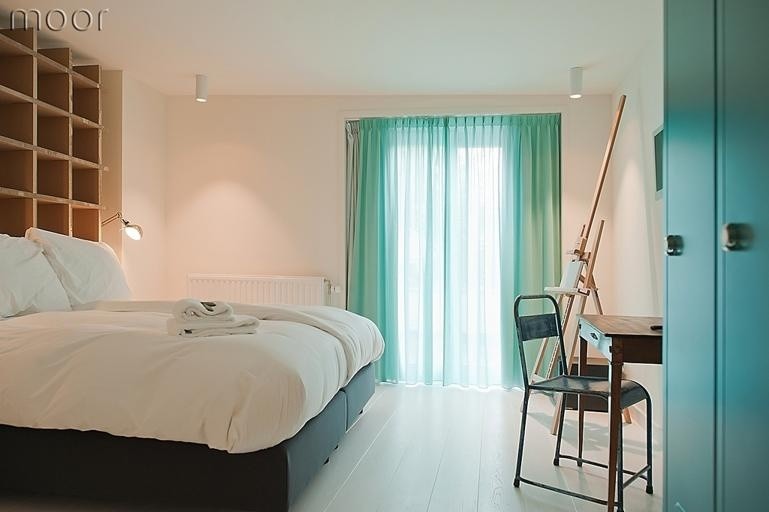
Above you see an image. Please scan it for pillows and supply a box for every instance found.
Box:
[0,227,133,318]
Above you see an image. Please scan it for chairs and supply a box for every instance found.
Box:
[512,293,654,512]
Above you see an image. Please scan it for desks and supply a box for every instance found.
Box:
[575,314,664,512]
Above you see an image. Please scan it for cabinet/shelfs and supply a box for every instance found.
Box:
[1,29,110,236]
[663,1,769,512]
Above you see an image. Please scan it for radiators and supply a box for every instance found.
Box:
[184,271,329,308]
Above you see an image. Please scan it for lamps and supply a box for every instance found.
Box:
[195,74,209,103]
[100,213,143,240]
[569,66,583,100]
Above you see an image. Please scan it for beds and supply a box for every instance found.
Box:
[0,298,388,512]
